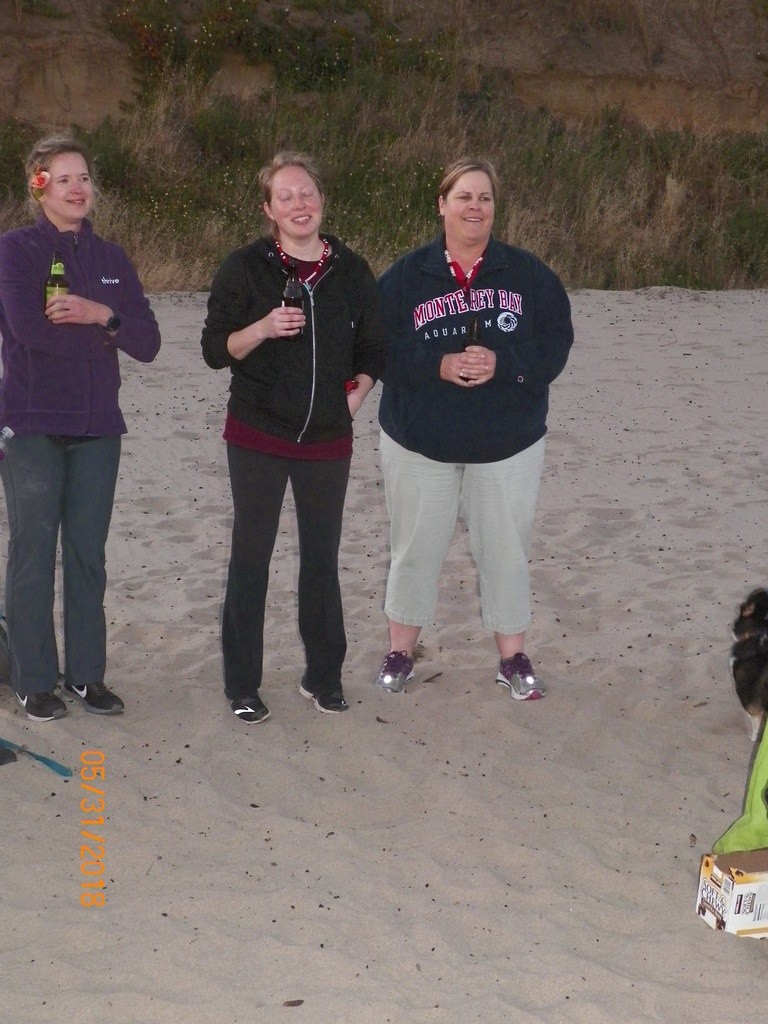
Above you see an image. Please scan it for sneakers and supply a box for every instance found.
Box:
[62,680,126,715]
[298,676,349,714]
[13,691,67,722]
[374,650,414,696]
[495,653,548,701]
[226,690,271,725]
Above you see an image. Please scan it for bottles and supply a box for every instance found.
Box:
[281,268,304,341]
[46,251,70,315]
[462,317,486,381]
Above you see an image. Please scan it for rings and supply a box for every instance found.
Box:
[459,371,465,378]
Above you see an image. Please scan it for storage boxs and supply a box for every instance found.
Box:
[696,846,768,943]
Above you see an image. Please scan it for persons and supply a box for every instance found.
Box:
[379,158,574,700]
[731,590,767,744]
[0,135,161,722]
[202,154,377,726]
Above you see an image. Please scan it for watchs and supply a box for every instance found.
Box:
[100,314,121,331]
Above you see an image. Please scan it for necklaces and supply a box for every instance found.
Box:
[274,239,328,283]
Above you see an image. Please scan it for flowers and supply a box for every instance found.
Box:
[31,173,50,189]
